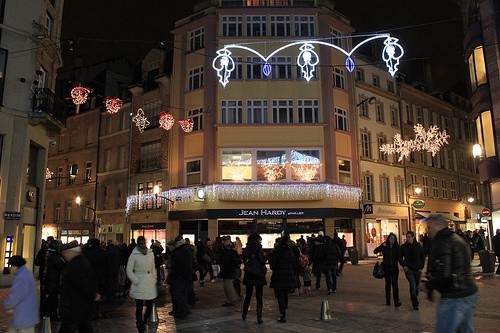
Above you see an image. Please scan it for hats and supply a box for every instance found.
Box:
[59,240,78,250]
[7,255,26,267]
[88,238,100,245]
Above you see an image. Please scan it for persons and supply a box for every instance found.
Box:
[267,236,295,324]
[170,236,193,318]
[417,224,500,282]
[56,239,104,332]
[240,231,267,326]
[125,231,158,332]
[321,235,342,296]
[399,230,424,310]
[32,234,165,320]
[422,213,481,333]
[172,229,348,311]
[3,254,42,333]
[372,231,404,309]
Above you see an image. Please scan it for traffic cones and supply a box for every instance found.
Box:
[320,301,334,322]
[240,296,251,312]
[150,303,157,322]
[42,317,51,333]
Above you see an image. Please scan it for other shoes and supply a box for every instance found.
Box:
[257,318,264,324]
[394,301,401,307]
[385,297,391,306]
[276,317,286,323]
[237,296,244,302]
[222,301,235,306]
[242,314,247,320]
[413,305,419,310]
[292,270,343,296]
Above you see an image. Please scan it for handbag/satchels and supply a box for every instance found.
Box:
[243,247,267,277]
[373,243,385,279]
[297,246,310,268]
[201,247,212,262]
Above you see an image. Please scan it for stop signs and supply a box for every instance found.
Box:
[482,208,490,216]
